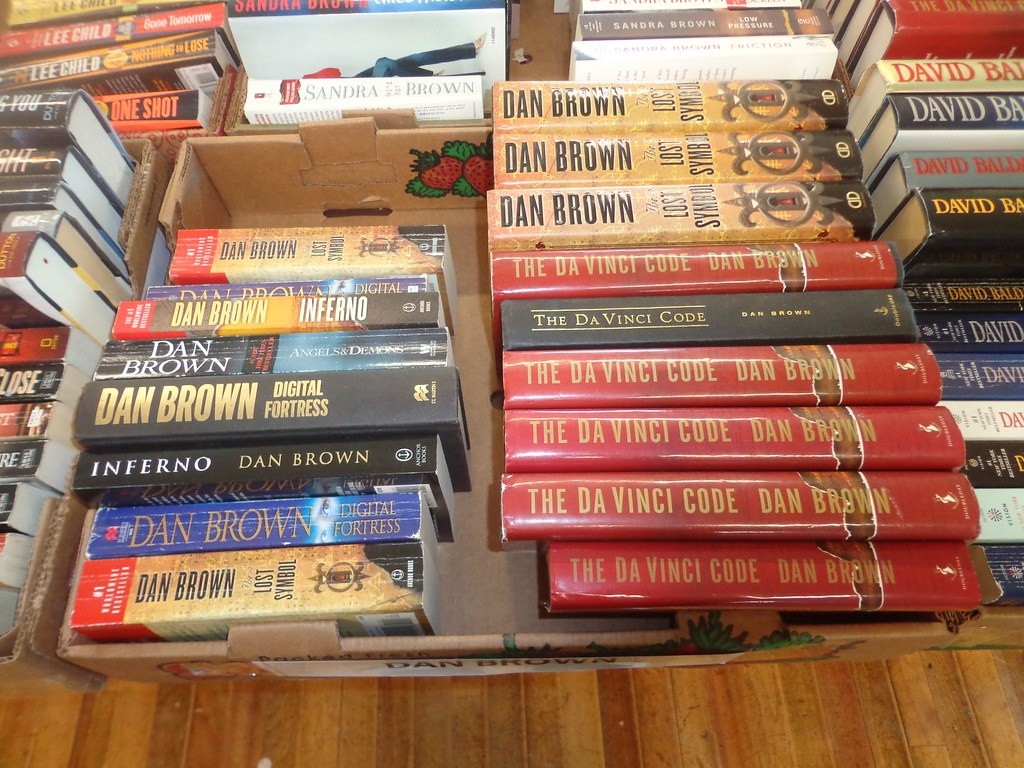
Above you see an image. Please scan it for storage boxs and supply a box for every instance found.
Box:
[224,0,856,137]
[1,138,174,694]
[55,113,959,687]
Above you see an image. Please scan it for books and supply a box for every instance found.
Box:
[551,0,840,84]
[811,1,1023,619]
[64,219,473,649]
[0,0,251,654]
[490,69,984,628]
[225,0,522,129]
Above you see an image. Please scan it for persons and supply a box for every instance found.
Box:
[302,33,487,79]
